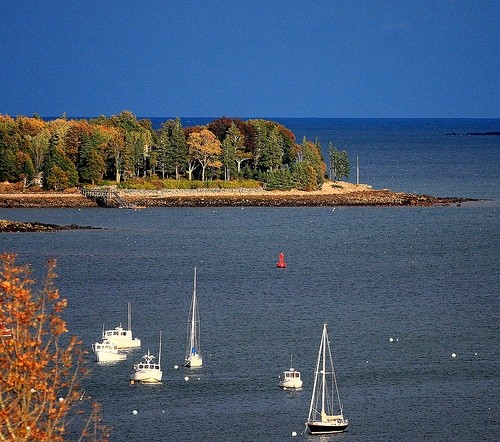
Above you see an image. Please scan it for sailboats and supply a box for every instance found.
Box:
[305,323,349,435]
[183,267,202,368]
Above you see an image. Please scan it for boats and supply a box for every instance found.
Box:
[132,330,164,385]
[278,354,303,389]
[91,302,142,364]
